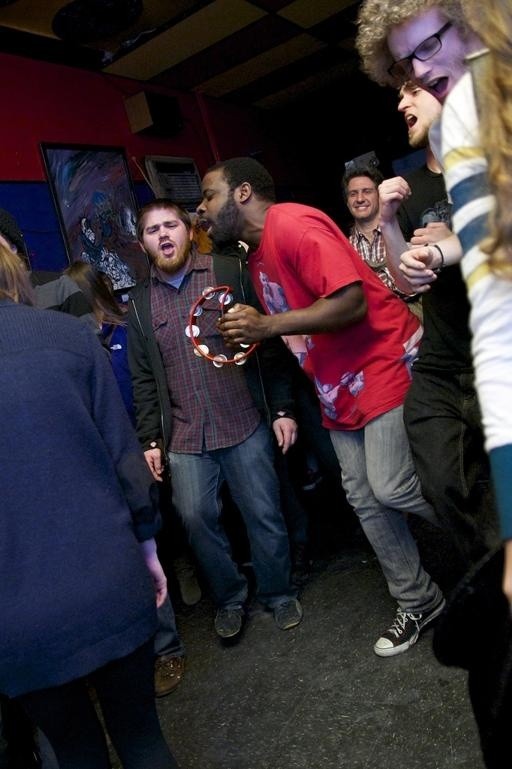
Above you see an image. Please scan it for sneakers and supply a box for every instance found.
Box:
[274,599,302,630]
[214,605,244,638]
[153,655,184,697]
[373,598,446,657]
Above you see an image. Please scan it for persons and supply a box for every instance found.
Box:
[0,233,205,767]
[375,77,499,669]
[125,153,444,658]
[354,0,487,178]
[434,1,511,608]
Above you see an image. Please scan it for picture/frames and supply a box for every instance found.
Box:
[37,139,151,301]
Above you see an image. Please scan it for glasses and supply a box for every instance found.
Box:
[387,22,454,80]
